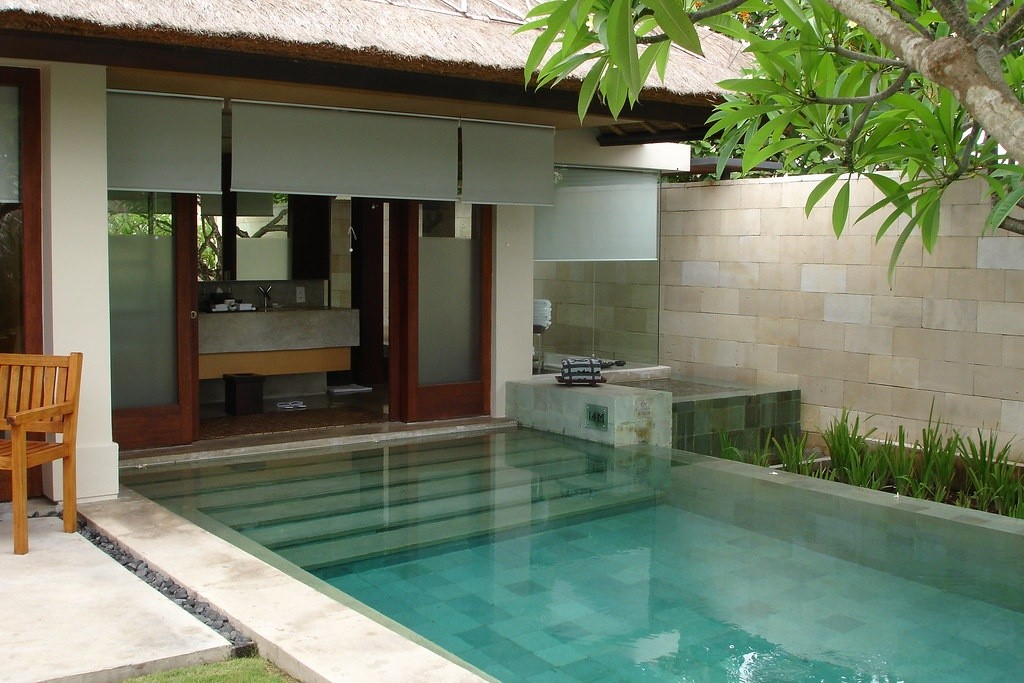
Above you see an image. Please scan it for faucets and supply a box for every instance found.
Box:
[258,286,273,307]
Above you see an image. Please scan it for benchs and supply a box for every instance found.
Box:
[0,352,84,555]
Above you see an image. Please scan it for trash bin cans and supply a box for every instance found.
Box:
[223,371,265,417]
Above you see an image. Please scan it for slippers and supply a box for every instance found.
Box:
[290,401,306,408]
[277,402,293,408]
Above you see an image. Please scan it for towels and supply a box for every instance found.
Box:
[533,297,554,331]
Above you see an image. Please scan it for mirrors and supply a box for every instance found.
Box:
[197,153,330,284]
